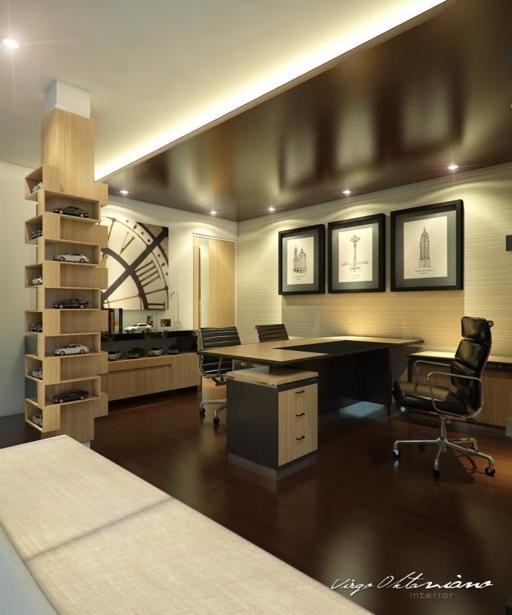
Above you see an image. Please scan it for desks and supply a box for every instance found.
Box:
[0,434,379,615]
[199,335,424,482]
[405,351,512,441]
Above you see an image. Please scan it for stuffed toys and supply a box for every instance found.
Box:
[53,253,90,263]
[32,275,42,285]
[31,228,42,238]
[51,390,88,403]
[32,414,42,426]
[32,368,42,379]
[53,207,88,218]
[31,182,42,192]
[125,322,152,334]
[31,320,42,332]
[108,345,180,360]
[53,298,88,309]
[54,344,89,356]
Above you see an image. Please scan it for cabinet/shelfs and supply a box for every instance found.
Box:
[104,332,199,402]
[23,108,110,443]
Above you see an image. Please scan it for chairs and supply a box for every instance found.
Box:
[200,326,253,425]
[394,316,495,479]
[255,324,289,342]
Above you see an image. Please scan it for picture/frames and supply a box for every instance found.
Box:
[277,223,326,295]
[327,212,386,293]
[389,198,465,292]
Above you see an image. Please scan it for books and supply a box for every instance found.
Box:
[109,308,123,333]
